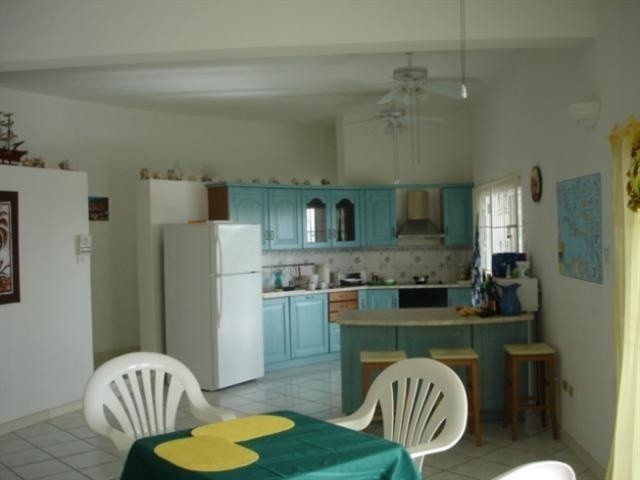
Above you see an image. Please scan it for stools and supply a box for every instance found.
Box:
[503,343,560,442]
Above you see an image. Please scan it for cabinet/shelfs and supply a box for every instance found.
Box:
[363,191,392,246]
[260,292,329,371]
[399,322,530,420]
[206,188,303,251]
[303,190,361,249]
[340,326,398,415]
[366,291,400,313]
[441,187,474,248]
[447,288,473,307]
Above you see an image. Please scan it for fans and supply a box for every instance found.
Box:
[334,51,483,136]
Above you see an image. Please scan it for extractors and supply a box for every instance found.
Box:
[396,190,447,239]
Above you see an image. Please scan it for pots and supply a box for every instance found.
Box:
[413,275,429,285]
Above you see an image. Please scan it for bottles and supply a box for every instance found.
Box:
[479,274,498,314]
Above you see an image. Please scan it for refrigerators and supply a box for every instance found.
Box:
[161,221,266,394]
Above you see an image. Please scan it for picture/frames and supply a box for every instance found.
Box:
[0,191,21,305]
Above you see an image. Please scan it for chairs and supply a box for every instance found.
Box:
[493,460,577,480]
[82,351,242,467]
[325,357,474,474]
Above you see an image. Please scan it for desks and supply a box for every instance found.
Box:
[120,411,426,480]
